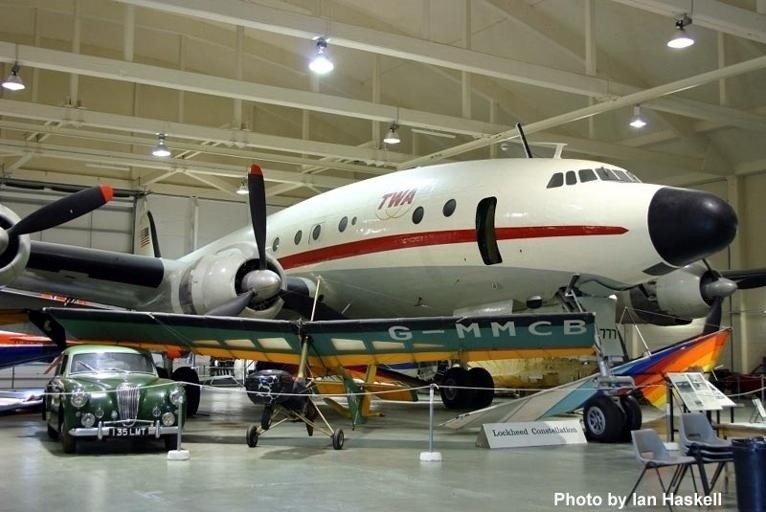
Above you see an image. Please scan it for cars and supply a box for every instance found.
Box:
[43,345,187,455]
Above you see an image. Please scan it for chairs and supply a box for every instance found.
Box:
[626,427,701,510]
[665,409,736,503]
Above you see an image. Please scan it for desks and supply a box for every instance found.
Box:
[715,421,766,495]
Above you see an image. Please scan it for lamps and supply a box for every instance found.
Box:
[0,54,27,92]
[665,11,697,52]
[629,102,647,130]
[235,173,252,196]
[149,131,173,158]
[382,118,403,146]
[305,33,335,77]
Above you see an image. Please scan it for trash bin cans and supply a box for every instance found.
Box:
[730,436,766,512]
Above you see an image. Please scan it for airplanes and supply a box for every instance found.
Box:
[0,149,766,439]
[38,300,600,449]
